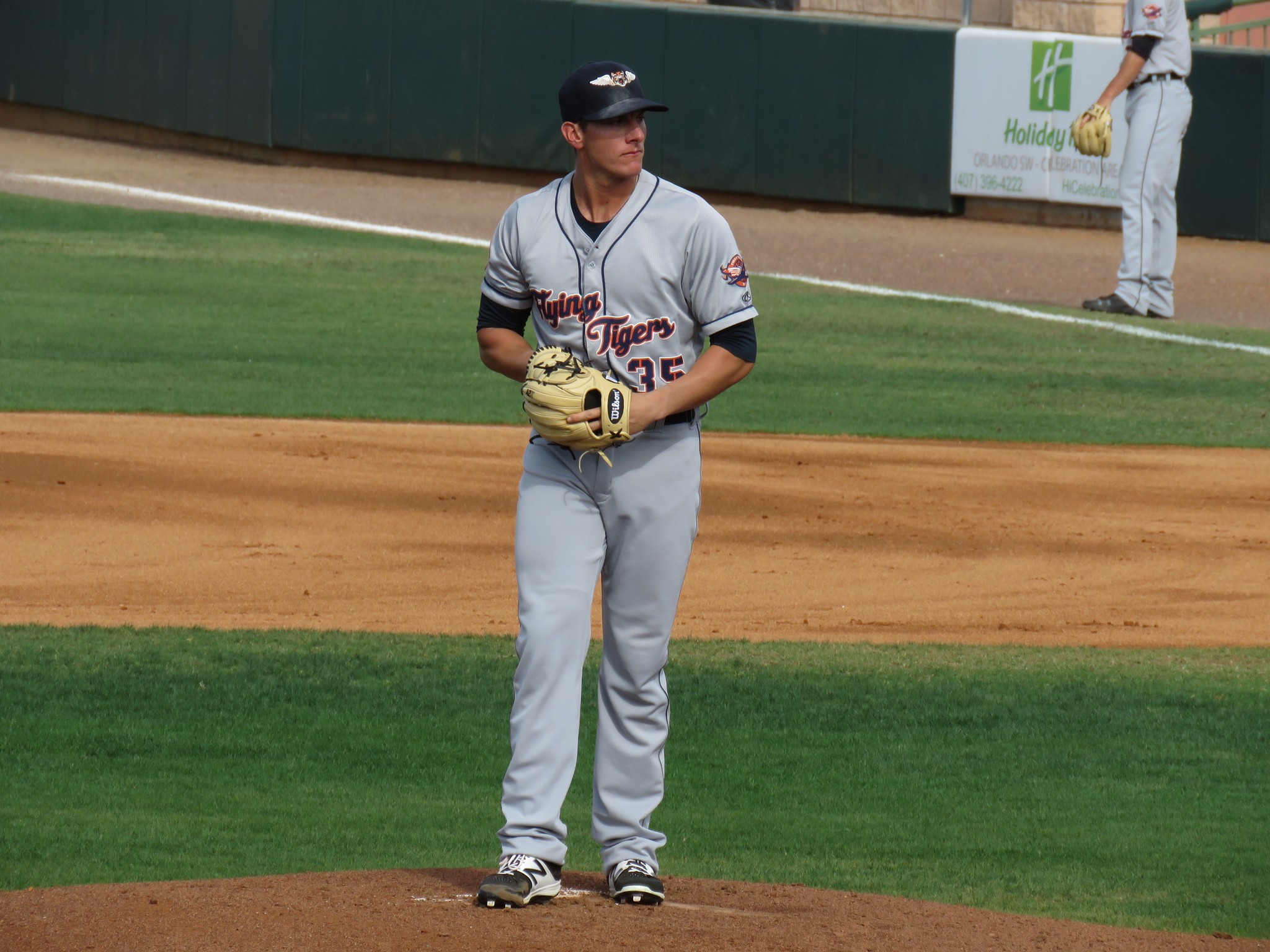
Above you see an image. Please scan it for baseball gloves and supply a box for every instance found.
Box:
[1070,103,1112,158]
[520,344,632,452]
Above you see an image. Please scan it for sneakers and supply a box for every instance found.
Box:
[607,860,664,905]
[1081,291,1143,317]
[1147,307,1169,318]
[474,854,563,909]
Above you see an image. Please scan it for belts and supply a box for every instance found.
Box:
[661,408,696,427]
[1126,70,1182,89]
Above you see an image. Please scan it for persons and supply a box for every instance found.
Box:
[1070,0,1192,318]
[478,62,758,905]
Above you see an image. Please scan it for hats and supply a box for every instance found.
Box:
[558,60,669,122]
